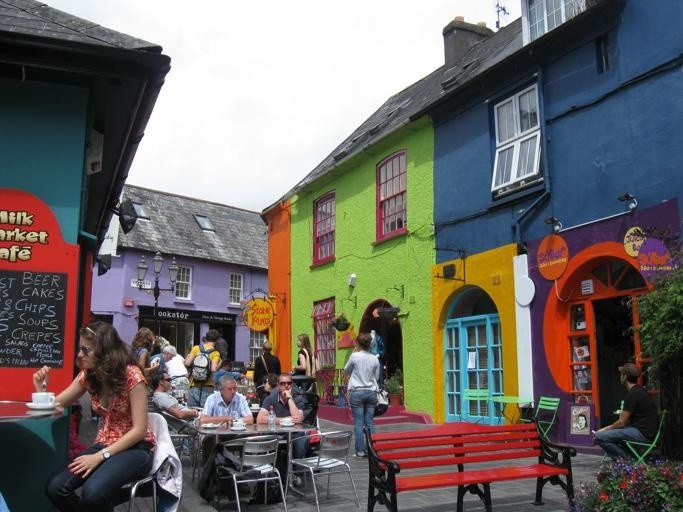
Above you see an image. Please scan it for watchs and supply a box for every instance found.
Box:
[101,447,111,459]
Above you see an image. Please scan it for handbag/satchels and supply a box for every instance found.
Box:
[374,392,388,416]
[254,481,279,503]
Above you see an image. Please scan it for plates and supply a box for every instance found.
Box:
[24,401,61,410]
[230,427,247,431]
[25,409,61,415]
[279,422,295,427]
[200,423,219,429]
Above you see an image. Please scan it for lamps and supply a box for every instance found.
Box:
[543,192,637,233]
[93,253,111,276]
[107,195,137,234]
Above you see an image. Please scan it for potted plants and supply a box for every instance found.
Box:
[384,377,403,407]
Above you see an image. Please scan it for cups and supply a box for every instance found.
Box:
[280,417,293,424]
[31,391,56,407]
[232,420,246,427]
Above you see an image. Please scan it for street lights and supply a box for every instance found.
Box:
[136,249,179,338]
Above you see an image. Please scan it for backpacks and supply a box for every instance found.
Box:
[192,344,216,381]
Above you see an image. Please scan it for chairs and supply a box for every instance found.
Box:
[108,411,164,512]
[285,427,360,512]
[191,417,203,481]
[458,388,489,425]
[210,431,284,512]
[160,409,196,467]
[598,401,668,465]
[519,396,560,450]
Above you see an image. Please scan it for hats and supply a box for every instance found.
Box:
[618,364,640,377]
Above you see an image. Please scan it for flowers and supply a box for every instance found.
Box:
[245,385,262,403]
[328,313,353,326]
[372,306,410,320]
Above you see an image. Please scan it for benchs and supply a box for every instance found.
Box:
[362,420,577,511]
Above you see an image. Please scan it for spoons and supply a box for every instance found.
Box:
[40,368,48,391]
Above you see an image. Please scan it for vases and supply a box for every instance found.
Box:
[333,322,350,331]
[373,310,396,324]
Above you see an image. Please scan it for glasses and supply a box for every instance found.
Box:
[163,378,172,382]
[279,382,292,386]
[78,346,92,354]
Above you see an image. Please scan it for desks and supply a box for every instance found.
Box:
[193,414,319,504]
[0,400,72,511]
[489,395,535,426]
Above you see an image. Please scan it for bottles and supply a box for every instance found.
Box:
[268,403,276,431]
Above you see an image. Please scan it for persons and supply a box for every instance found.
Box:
[343,333,380,457]
[31,320,155,512]
[591,363,658,462]
[577,414,588,429]
[129,326,320,500]
[368,329,385,384]
